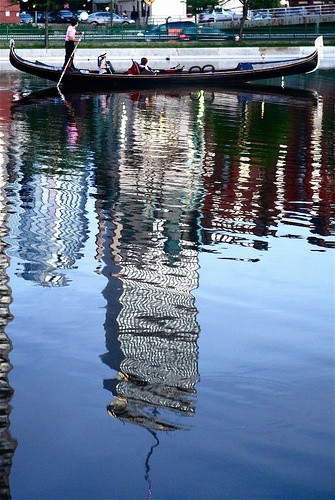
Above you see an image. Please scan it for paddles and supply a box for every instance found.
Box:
[56,33,84,87]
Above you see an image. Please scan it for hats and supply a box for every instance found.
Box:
[100,51,107,57]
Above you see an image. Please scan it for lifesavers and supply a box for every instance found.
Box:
[201,64,215,72]
[188,66,201,73]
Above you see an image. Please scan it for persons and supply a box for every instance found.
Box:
[97,51,114,74]
[61,18,79,71]
[139,57,150,71]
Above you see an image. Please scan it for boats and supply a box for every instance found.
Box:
[8,36,324,92]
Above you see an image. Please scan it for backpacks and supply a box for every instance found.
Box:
[128,61,141,75]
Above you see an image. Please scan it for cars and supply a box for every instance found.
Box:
[19,9,89,24]
[80,11,136,28]
[137,20,229,41]
[197,8,309,23]
